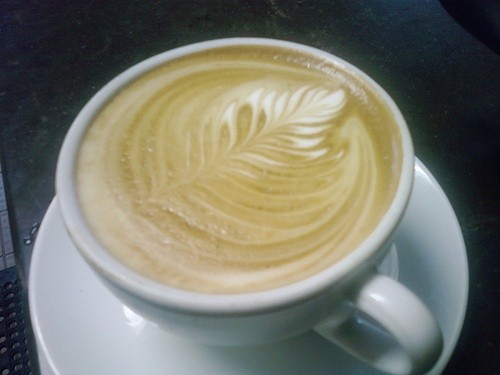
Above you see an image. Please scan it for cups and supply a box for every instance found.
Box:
[54,37,444,375]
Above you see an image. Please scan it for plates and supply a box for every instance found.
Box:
[27,155,471,375]
